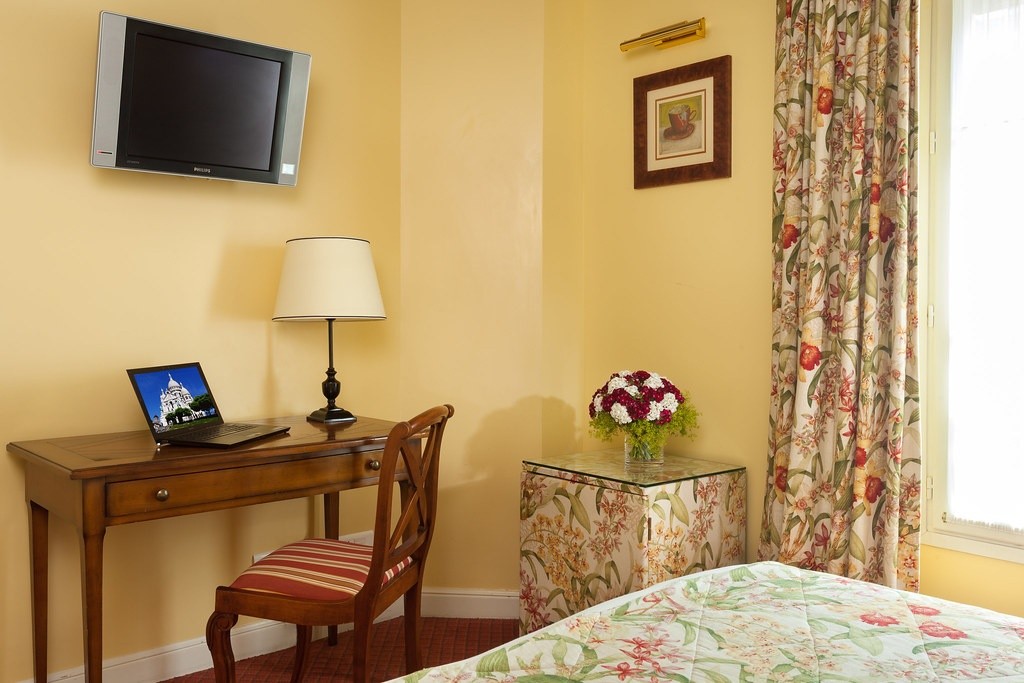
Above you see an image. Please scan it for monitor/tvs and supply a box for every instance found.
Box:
[89,11,312,188]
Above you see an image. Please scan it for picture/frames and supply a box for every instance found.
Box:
[633,54,732,190]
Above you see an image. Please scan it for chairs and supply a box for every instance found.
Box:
[205,403,456,683]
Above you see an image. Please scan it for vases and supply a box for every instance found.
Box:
[624,432,664,473]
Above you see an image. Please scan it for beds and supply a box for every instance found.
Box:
[376,558,1023,683]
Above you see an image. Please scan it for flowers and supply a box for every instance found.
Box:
[588,370,700,461]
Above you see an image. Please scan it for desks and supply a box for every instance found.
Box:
[4,408,431,682]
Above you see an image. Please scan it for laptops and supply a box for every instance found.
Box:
[126,362,291,449]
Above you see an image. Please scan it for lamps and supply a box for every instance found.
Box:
[272,236,389,424]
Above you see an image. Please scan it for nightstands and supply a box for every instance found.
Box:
[517,446,746,636]
